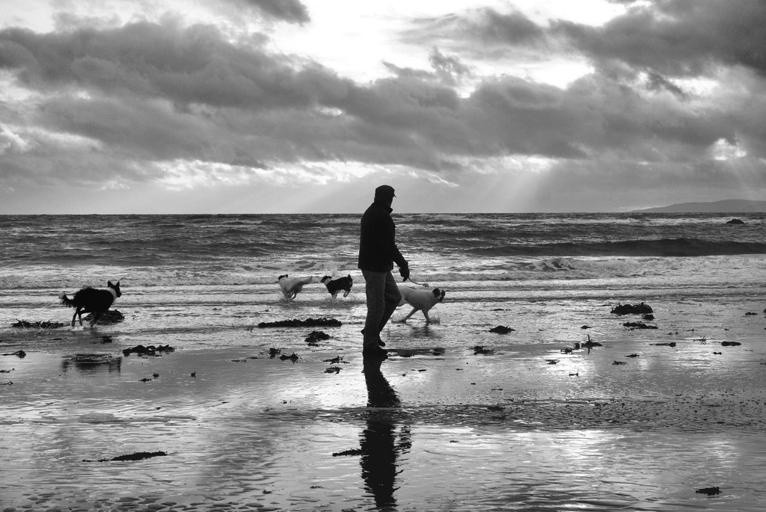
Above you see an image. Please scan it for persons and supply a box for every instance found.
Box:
[360,366,399,512]
[358,183,412,367]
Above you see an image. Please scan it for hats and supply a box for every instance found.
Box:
[375,185,397,197]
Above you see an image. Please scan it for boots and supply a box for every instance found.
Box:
[360,328,388,357]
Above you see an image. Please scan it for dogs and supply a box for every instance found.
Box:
[276,272,314,300]
[59,280,122,328]
[397,284,445,324]
[319,274,353,301]
